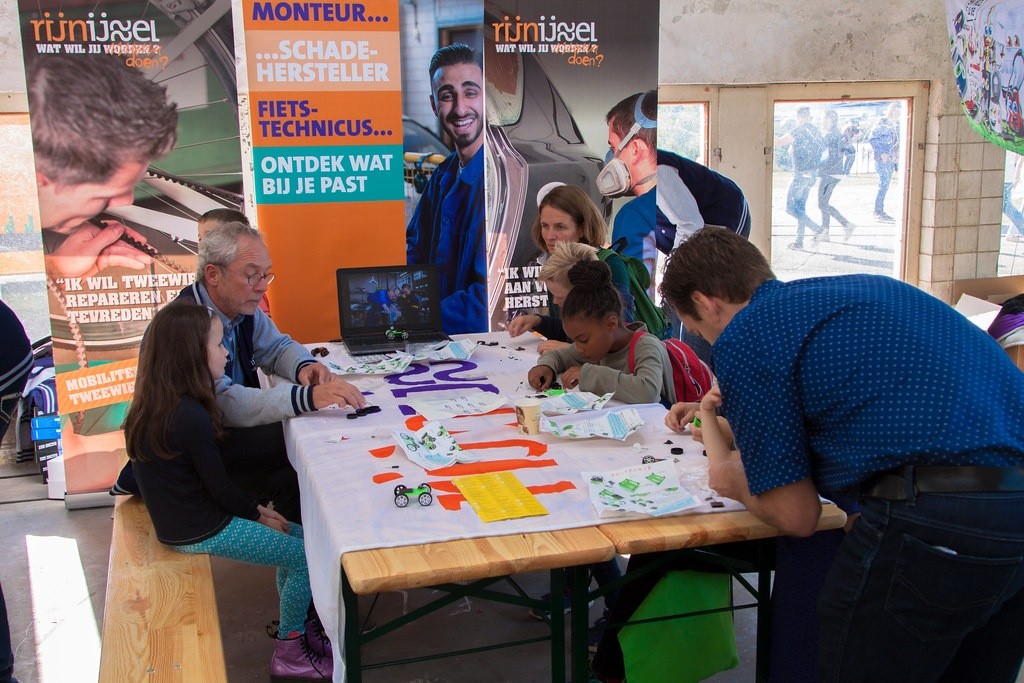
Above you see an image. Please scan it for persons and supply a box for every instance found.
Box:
[196,207,271,316]
[596,91,658,298]
[367,284,419,326]
[26,32,178,292]
[121,302,334,683]
[814,111,857,242]
[774,106,828,250]
[108,221,367,526]
[528,258,677,651]
[0,300,34,452]
[406,45,489,333]
[508,242,599,344]
[532,184,636,333]
[1003,149,1024,236]
[659,225,1024,683]
[868,101,901,222]
[655,148,752,259]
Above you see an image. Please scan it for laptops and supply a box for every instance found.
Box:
[337,264,456,356]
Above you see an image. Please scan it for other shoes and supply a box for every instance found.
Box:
[529,587,595,620]
[843,222,856,241]
[812,228,828,244]
[874,212,894,224]
[588,609,610,652]
[787,243,803,250]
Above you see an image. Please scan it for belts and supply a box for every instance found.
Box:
[859,466,1024,501]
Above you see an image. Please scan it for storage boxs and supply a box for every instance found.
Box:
[31,406,67,499]
[954,275,1024,373]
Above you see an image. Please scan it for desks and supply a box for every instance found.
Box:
[257,331,847,683]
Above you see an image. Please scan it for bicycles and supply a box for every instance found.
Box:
[18,1,249,354]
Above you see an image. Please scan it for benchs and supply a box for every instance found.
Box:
[98,451,227,683]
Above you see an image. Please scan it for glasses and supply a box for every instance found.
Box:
[203,263,276,287]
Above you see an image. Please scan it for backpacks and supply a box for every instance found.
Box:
[623,322,714,404]
[596,238,674,341]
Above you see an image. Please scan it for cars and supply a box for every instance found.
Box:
[401,113,454,196]
[483,0,614,329]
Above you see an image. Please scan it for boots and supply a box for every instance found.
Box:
[266,625,333,683]
[304,619,333,657]
